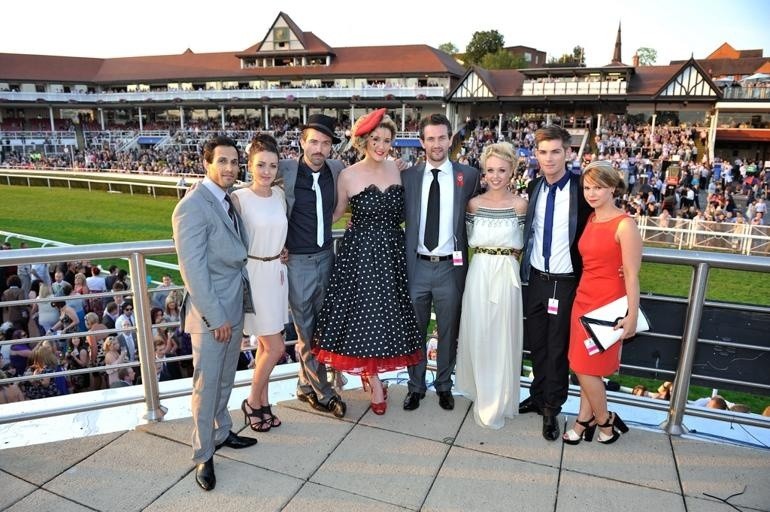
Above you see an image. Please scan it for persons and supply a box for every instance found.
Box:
[562,161,642,445]
[0,371,24,405]
[62,284,86,332]
[428,349,438,361]
[455,141,528,429]
[74,273,88,294]
[568,112,620,173]
[154,340,181,379]
[65,338,91,393]
[52,272,71,297]
[48,263,60,283]
[18,242,32,299]
[529,77,628,83]
[115,302,135,328]
[101,303,118,336]
[28,279,47,337]
[2,243,18,300]
[151,274,178,312]
[3,275,28,327]
[151,309,164,335]
[103,337,127,386]
[1,82,439,95]
[32,284,59,334]
[346,114,485,411]
[621,108,770,248]
[154,319,178,357]
[10,340,68,394]
[77,261,92,277]
[719,81,770,97]
[136,360,169,385]
[0,354,6,377]
[237,336,256,371]
[172,331,195,377]
[606,379,770,417]
[172,137,258,491]
[185,114,409,418]
[103,283,124,308]
[163,295,181,321]
[102,290,126,318]
[110,366,135,389]
[86,266,107,320]
[46,301,82,336]
[25,348,58,400]
[457,114,507,189]
[31,263,51,294]
[507,114,559,200]
[0,108,425,198]
[106,265,118,290]
[64,261,77,288]
[3,363,18,378]
[116,269,129,290]
[230,134,290,432]
[117,320,138,362]
[10,330,33,376]
[519,126,625,442]
[84,312,110,390]
[311,108,425,415]
[0,322,13,334]
[1,328,16,367]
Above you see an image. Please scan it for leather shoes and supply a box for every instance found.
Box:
[196,456,216,491]
[327,394,346,418]
[403,391,426,410]
[542,410,560,441]
[436,390,454,410]
[216,430,257,449]
[297,392,328,412]
[518,396,540,415]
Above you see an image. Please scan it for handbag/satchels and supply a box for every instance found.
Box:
[579,296,654,353]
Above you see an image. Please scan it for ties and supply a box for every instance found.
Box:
[423,168,441,253]
[542,185,557,258]
[225,194,239,236]
[311,173,325,248]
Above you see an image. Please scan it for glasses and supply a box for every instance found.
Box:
[125,308,132,312]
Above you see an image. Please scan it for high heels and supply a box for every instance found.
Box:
[360,373,387,400]
[371,389,386,415]
[241,399,271,433]
[261,403,282,427]
[595,411,629,444]
[561,416,597,446]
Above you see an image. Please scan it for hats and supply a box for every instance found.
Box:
[299,114,342,145]
[354,109,386,136]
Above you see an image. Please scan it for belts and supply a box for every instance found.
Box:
[417,253,453,263]
[530,264,576,282]
